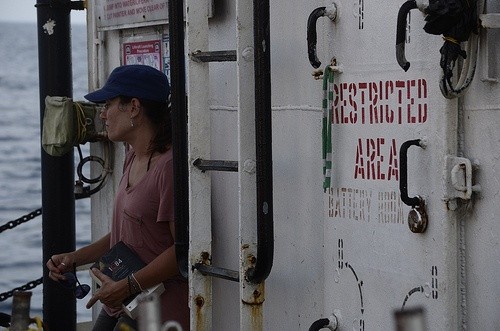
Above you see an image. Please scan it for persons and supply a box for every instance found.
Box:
[45,64,190,331]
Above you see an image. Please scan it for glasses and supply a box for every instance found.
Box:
[49,256,91,300]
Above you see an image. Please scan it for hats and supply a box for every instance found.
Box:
[83,63,171,108]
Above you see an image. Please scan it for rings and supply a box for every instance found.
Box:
[61,262,67,268]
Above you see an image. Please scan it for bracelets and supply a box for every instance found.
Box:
[126,271,144,297]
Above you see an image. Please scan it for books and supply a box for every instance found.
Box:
[90,241,167,320]
